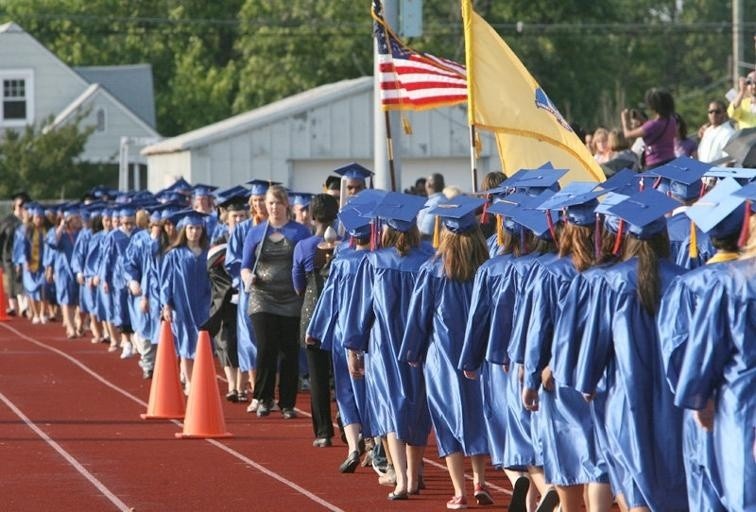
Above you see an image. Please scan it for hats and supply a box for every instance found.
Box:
[166,209,211,226]
[479,161,682,240]
[191,182,216,198]
[683,177,750,239]
[333,161,377,181]
[245,177,282,195]
[21,178,191,219]
[210,183,251,211]
[730,177,756,215]
[335,187,430,239]
[286,190,316,205]
[703,164,756,189]
[633,154,715,199]
[428,192,491,235]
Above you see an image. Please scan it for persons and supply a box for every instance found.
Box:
[0,68,755,511]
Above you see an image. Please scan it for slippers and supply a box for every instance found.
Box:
[386,487,410,501]
[406,476,421,495]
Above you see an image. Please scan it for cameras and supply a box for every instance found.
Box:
[745,78,753,86]
[629,110,637,119]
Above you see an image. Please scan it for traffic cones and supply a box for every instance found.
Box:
[174,331,235,439]
[139,321,187,420]
[0,266,12,322]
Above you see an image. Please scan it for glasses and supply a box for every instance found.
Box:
[708,109,724,114]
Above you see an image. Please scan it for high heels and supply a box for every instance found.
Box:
[360,448,373,468]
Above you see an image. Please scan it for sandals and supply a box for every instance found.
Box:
[225,389,238,403]
[237,390,248,402]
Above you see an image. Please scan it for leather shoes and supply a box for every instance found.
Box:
[337,450,362,474]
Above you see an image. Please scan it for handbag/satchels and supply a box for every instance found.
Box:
[640,148,646,167]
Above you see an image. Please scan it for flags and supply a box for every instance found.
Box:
[462,0,607,188]
[371,0,467,136]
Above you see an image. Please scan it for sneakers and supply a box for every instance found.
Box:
[118,340,132,359]
[38,313,49,325]
[107,338,118,352]
[246,398,259,413]
[63,322,75,339]
[507,476,531,511]
[48,312,56,321]
[100,333,110,342]
[377,469,397,487]
[137,359,146,370]
[179,370,187,385]
[5,307,17,317]
[534,487,560,512]
[31,313,39,325]
[371,458,389,476]
[256,403,271,417]
[280,406,298,419]
[142,366,153,380]
[76,323,85,338]
[183,381,192,395]
[444,494,470,509]
[473,484,496,505]
[312,436,331,448]
[90,335,102,344]
